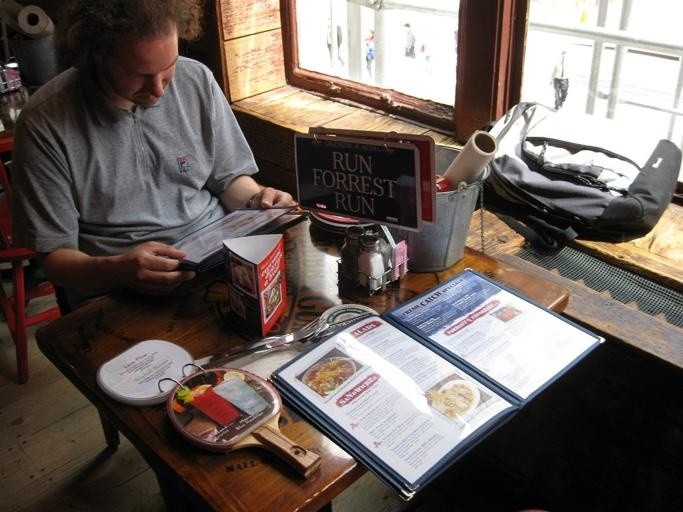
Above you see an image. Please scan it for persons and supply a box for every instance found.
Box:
[550,51,569,112]
[405,24,417,61]
[327,17,345,67]
[365,29,375,78]
[12,0,300,307]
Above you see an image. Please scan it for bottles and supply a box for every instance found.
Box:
[342,227,394,290]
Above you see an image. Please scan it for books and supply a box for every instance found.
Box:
[266,266,606,504]
[168,206,309,274]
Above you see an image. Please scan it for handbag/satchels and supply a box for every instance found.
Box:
[475,101,682,243]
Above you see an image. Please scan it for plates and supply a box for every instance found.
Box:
[300,357,357,391]
[430,379,482,416]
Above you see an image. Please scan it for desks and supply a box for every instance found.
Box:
[36,245,572,510]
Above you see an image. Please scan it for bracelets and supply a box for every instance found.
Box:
[246,193,260,208]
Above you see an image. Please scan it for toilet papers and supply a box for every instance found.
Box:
[18,6,56,37]
[444,129,497,190]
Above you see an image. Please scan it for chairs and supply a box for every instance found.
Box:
[0,135,62,385]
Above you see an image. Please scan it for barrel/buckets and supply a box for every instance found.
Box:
[7,33,77,97]
[382,145,492,273]
